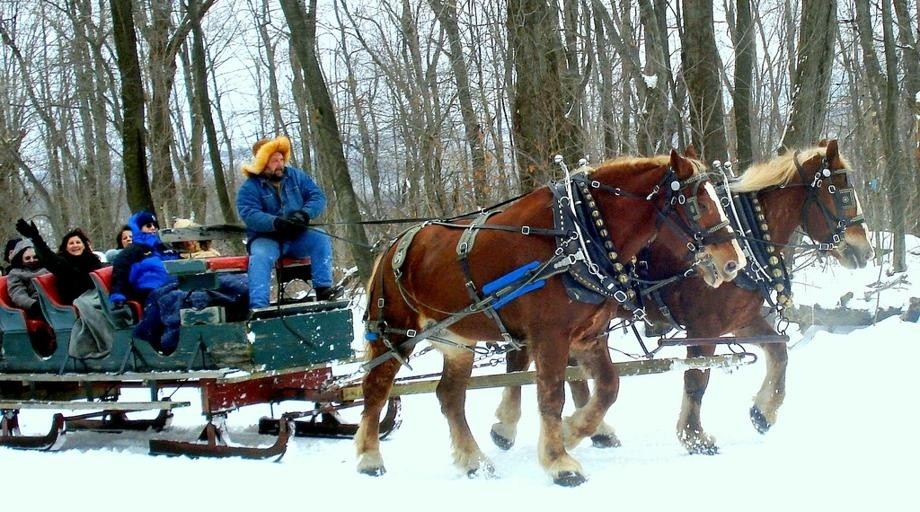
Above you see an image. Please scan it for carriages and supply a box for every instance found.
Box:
[0,139,874,487]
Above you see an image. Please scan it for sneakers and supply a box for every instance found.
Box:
[315,284,345,302]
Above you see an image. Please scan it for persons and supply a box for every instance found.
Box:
[6,237,56,357]
[14,217,102,307]
[171,217,251,322]
[236,135,344,314]
[108,207,215,355]
[105,224,133,266]
[2,237,25,277]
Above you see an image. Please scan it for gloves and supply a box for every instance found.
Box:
[272,209,310,241]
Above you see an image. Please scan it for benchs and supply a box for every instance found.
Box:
[1,227,312,358]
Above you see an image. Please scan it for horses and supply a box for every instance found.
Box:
[353,144,747,487]
[490,139,874,455]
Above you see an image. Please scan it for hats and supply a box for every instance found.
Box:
[239,135,292,178]
[8,237,36,261]
[135,210,158,231]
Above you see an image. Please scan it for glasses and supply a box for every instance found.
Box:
[145,223,159,228]
[24,254,38,261]
